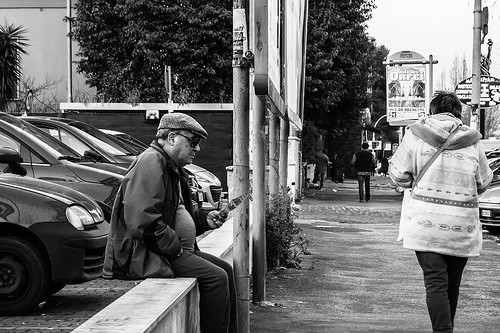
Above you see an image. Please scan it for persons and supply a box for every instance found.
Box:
[351,143,378,203]
[390,92,493,333]
[381,154,389,177]
[101,113,238,333]
[313,149,340,191]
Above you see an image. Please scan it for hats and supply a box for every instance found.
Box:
[158,113,208,140]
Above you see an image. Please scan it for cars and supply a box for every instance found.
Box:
[0,111,132,225]
[477,147,500,234]
[0,172,112,315]
[49,125,224,212]
[14,116,205,223]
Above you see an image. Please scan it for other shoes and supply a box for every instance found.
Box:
[359,200,363,203]
[365,199,369,202]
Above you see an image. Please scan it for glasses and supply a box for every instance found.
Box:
[174,133,200,148]
